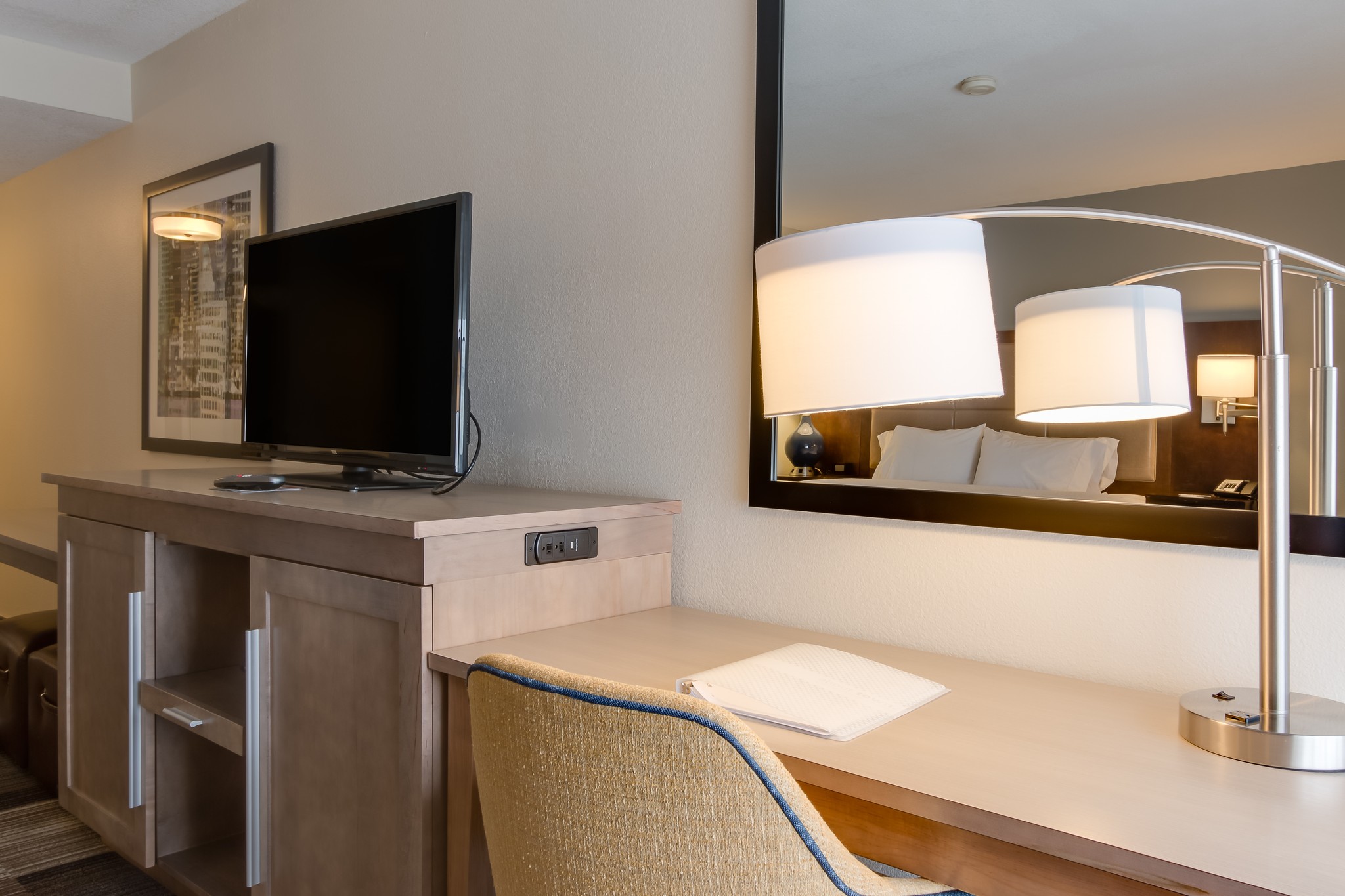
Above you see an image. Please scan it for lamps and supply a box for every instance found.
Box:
[1014,257,1338,518]
[784,414,823,476]
[755,208,1344,772]
[152,211,222,244]
[1197,353,1258,436]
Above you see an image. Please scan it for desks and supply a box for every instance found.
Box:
[425,602,1345,896]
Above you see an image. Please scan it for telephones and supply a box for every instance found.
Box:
[1213,479,1258,499]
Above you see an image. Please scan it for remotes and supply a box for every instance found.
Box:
[214,474,287,490]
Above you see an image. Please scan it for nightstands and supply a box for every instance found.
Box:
[1145,488,1256,510]
[775,474,847,482]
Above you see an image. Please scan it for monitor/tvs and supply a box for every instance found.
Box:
[243,190,472,493]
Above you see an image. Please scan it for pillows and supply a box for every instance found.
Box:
[871,422,987,484]
[973,426,1120,495]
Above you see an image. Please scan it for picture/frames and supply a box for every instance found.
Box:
[138,143,277,461]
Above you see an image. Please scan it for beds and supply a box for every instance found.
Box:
[859,332,1172,505]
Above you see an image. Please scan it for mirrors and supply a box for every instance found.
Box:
[748,2,1345,557]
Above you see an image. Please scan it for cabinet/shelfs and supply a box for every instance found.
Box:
[56,484,153,877]
[243,515,675,896]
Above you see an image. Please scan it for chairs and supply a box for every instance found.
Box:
[466,652,969,896]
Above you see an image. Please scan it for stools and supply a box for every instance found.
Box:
[1,608,56,769]
[25,642,57,794]
